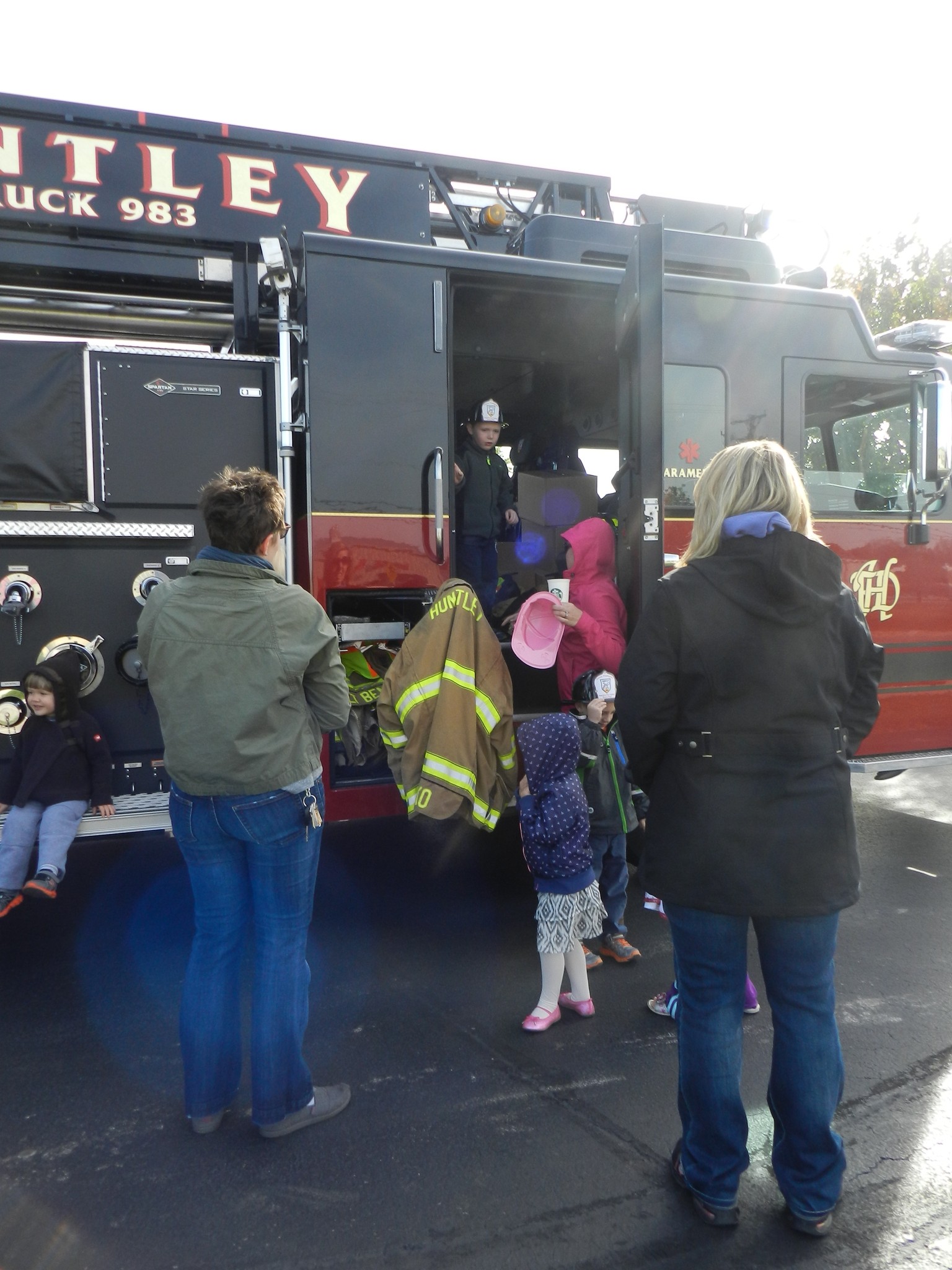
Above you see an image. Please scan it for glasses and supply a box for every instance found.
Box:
[276,521,290,539]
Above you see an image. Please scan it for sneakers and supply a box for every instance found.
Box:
[0,888,23,917]
[599,932,642,963]
[259,1083,351,1139]
[789,1177,844,1235]
[669,1138,739,1223]
[192,1107,223,1134]
[582,944,603,970]
[22,873,57,898]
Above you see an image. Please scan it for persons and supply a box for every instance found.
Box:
[454,397,518,621]
[500,438,887,1239]
[137,466,351,1140]
[0,650,117,919]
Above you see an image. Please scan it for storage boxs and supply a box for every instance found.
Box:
[494,469,602,614]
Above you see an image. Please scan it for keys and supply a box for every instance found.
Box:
[304,808,311,843]
[309,802,322,829]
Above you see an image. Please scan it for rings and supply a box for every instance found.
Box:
[566,612,568,617]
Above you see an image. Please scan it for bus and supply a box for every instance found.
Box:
[1,89,951,850]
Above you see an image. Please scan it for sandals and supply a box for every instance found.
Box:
[744,1004,760,1013]
[647,992,671,1016]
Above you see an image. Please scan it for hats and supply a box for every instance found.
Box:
[572,670,618,702]
[512,592,566,668]
[465,398,503,423]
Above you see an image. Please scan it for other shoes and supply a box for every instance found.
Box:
[522,1005,561,1032]
[558,992,595,1017]
